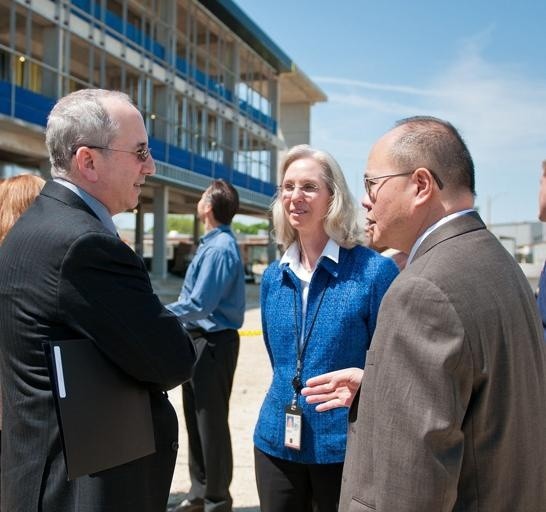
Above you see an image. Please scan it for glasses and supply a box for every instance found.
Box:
[72,144,152,161]
[363,168,443,202]
[274,182,326,197]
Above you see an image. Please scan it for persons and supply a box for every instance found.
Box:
[1,174,45,249]
[533,159,546,325]
[296,113,546,509]
[361,222,412,273]
[2,84,202,511]
[161,174,246,512]
[248,142,400,512]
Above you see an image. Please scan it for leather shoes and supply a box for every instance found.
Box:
[165,498,203,512]
[202,498,232,512]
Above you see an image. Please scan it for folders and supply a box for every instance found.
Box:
[42,338,156,481]
